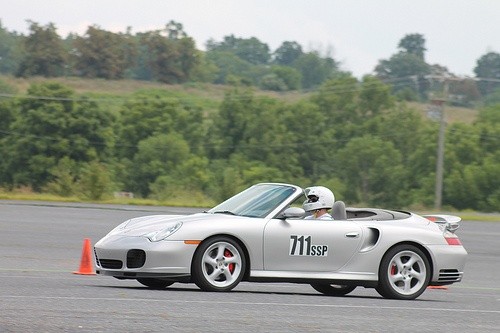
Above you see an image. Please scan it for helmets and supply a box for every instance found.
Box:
[302,186,334,212]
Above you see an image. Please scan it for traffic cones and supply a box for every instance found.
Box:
[74,238,97,276]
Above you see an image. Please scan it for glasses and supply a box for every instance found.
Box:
[308,197,317,203]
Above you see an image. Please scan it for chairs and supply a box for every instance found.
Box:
[331,201,347,220]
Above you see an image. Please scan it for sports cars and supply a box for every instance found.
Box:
[93,181,468,302]
